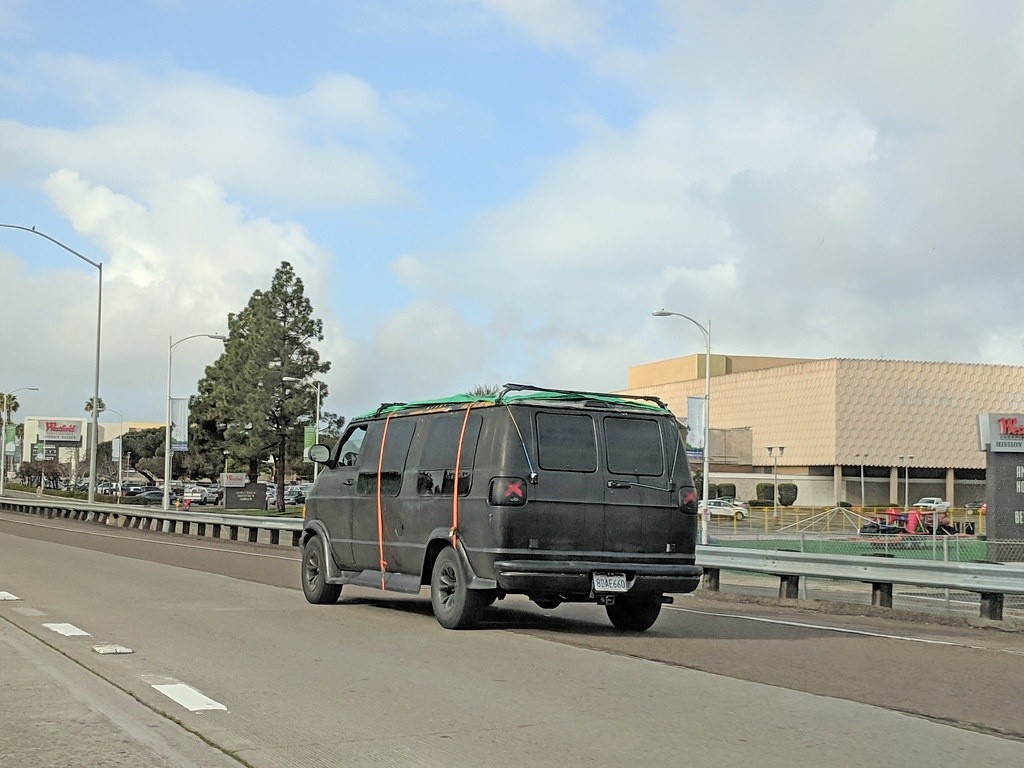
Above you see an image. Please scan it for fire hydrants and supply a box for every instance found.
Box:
[174,498,181,511]
[183,498,191,511]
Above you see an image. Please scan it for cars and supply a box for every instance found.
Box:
[964,502,986,514]
[719,495,749,508]
[25,474,313,505]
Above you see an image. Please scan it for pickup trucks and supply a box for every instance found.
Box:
[913,498,952,516]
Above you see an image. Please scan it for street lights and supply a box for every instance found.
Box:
[283,376,321,484]
[653,309,713,546]
[855,453,871,505]
[897,455,914,510]
[764,445,786,515]
[96,406,122,497]
[162,333,226,509]
[0,386,40,497]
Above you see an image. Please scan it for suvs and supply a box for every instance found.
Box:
[295,380,702,635]
[693,499,750,521]
